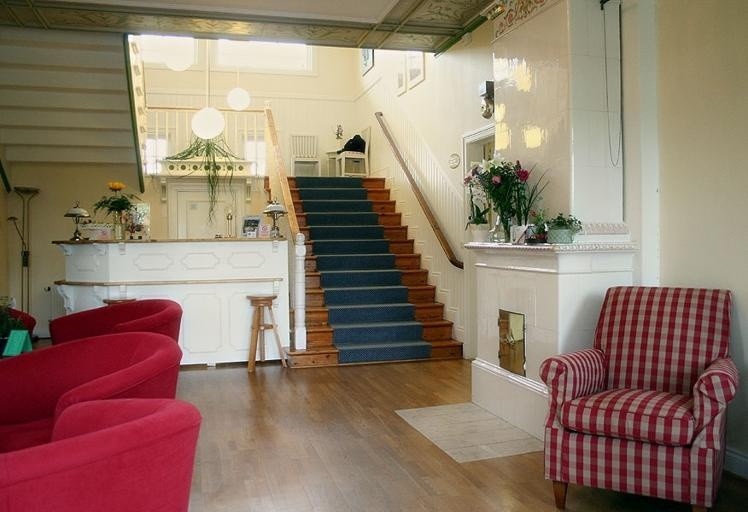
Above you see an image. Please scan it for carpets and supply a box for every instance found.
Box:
[392,400,545,464]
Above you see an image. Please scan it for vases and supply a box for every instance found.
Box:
[508,224,528,245]
[110,221,126,243]
[544,228,574,245]
[469,223,490,244]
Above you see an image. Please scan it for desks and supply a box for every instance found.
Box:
[325,150,363,177]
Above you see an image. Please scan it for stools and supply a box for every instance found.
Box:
[245,293,288,375]
[102,298,136,304]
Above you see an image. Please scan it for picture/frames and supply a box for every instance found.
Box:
[359,48,375,78]
[407,51,426,91]
[239,214,265,237]
[394,64,406,97]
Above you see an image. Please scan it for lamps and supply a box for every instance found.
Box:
[226,40,252,113]
[191,38,225,142]
[263,196,289,238]
[63,199,89,243]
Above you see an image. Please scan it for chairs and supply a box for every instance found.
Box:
[1,305,37,336]
[290,133,322,177]
[0,330,183,453]
[0,397,203,511]
[49,298,183,346]
[538,285,741,511]
[335,126,373,178]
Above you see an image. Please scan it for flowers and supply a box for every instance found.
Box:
[463,156,583,239]
[90,177,143,229]
